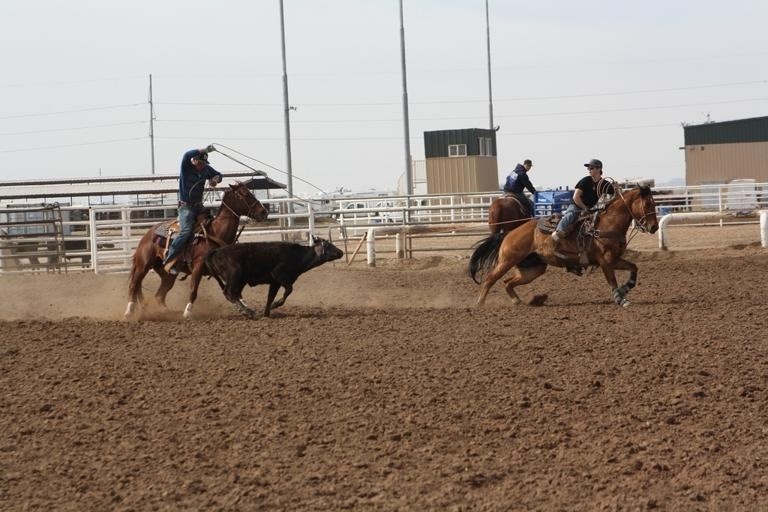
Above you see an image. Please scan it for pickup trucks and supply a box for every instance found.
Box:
[335,201,372,218]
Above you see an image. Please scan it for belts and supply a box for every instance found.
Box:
[178,200,203,206]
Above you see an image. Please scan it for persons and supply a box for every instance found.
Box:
[158,145,224,276]
[502,159,537,218]
[550,160,614,242]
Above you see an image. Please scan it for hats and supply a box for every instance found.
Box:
[194,153,210,165]
[584,159,603,167]
[523,159,534,166]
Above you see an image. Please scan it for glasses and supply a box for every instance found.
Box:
[587,167,595,169]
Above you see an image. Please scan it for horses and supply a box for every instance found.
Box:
[124,178,270,320]
[487,193,534,235]
[468,182,659,308]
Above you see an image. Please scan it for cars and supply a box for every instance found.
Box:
[374,201,392,219]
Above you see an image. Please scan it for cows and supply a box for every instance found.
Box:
[205,234,344,320]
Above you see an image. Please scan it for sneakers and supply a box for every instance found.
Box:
[161,260,179,276]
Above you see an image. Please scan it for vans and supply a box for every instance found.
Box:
[725,177,768,217]
